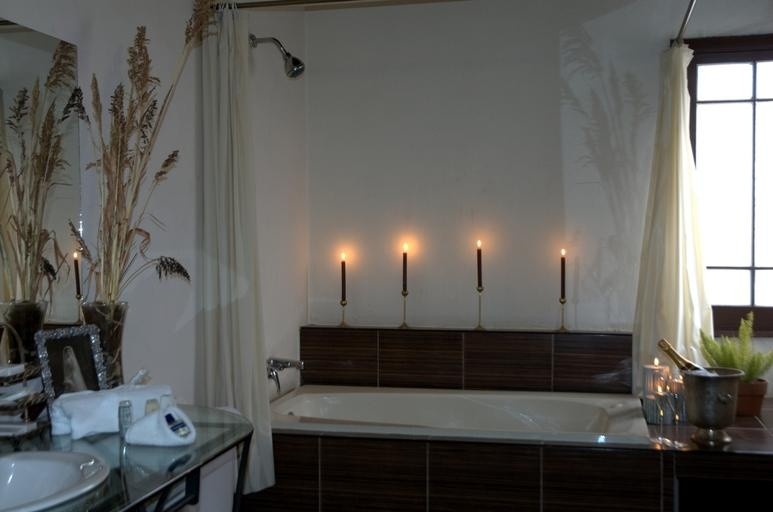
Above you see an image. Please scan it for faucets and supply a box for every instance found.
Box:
[267,356,307,391]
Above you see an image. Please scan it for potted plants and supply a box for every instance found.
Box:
[699,312,773,417]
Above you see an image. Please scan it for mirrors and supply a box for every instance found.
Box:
[0,16,83,368]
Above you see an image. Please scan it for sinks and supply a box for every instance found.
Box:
[1,449,113,511]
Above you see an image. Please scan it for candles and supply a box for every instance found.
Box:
[477,239,482,287]
[73,252,80,294]
[561,248,565,298]
[402,243,407,292]
[341,253,346,300]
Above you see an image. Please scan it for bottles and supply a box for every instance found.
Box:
[144,400,159,415]
[118,401,132,435]
[656,339,712,374]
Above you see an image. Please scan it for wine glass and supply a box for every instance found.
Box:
[639,367,743,450]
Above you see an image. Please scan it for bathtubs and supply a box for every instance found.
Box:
[270,382,652,442]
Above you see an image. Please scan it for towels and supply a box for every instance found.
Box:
[52,386,173,441]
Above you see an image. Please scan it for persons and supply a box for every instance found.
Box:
[63,346,88,391]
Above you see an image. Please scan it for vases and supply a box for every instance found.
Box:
[0,301,50,366]
[68,302,128,389]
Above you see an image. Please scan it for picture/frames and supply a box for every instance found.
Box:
[34,324,107,414]
